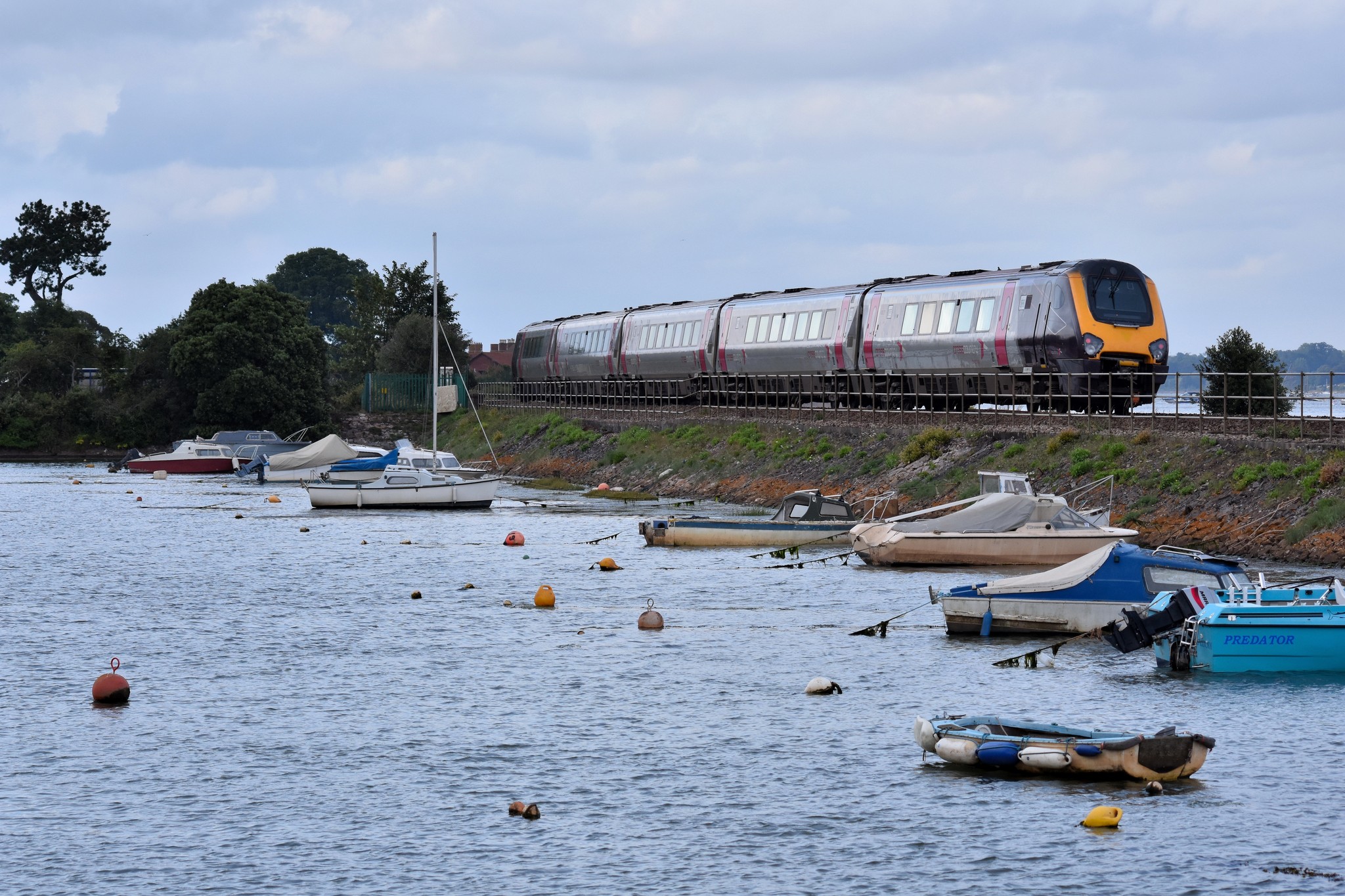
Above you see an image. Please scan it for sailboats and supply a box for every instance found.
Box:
[299,230,505,513]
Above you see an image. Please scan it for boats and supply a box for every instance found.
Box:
[848,469,1141,569]
[319,437,493,485]
[107,428,390,485]
[1143,567,1345,678]
[912,710,1218,784]
[637,488,899,548]
[928,534,1258,640]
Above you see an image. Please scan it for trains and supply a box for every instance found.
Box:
[508,254,1173,417]
